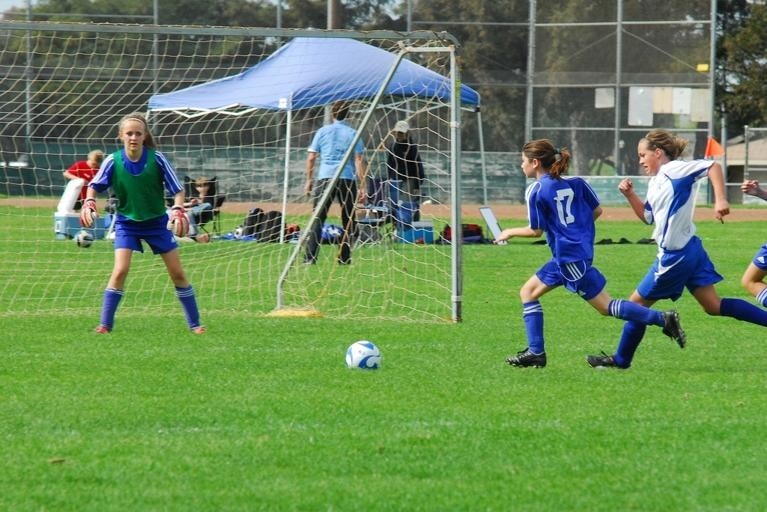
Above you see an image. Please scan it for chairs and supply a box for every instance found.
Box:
[167,176,226,241]
[367,178,387,225]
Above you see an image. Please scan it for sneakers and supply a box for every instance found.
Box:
[506,347,547,369]
[585,351,614,368]
[196,234,210,243]
[662,311,686,348]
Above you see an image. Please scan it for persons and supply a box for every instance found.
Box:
[738,178,767,311]
[304,100,369,265]
[62,149,104,206]
[585,131,767,368]
[387,120,424,222]
[493,136,686,366]
[184,178,214,242]
[81,112,206,333]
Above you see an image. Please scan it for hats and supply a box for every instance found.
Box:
[392,121,408,134]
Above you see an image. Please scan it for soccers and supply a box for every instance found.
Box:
[76,231,96,247]
[234,225,246,240]
[346,339,381,370]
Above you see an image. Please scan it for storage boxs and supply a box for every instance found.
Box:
[54,212,105,241]
[397,220,434,244]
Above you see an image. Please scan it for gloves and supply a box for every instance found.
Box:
[167,204,190,236]
[79,197,99,228]
